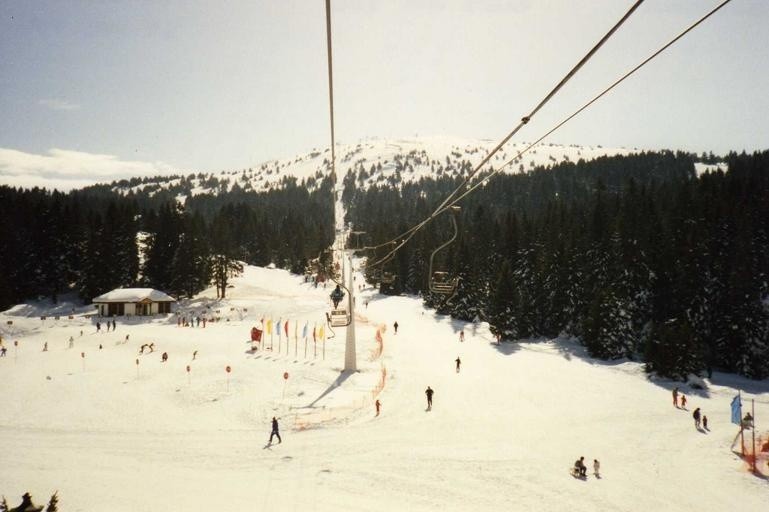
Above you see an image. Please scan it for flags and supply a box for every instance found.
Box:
[249,312,325,344]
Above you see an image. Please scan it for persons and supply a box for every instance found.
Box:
[330,283,344,310]
[681,395,686,408]
[574,456,587,476]
[68,335,75,348]
[593,458,601,477]
[393,321,399,333]
[268,415,282,444]
[672,387,679,406]
[703,415,707,427]
[692,408,701,428]
[0,346,8,357]
[425,386,434,408]
[454,356,462,372]
